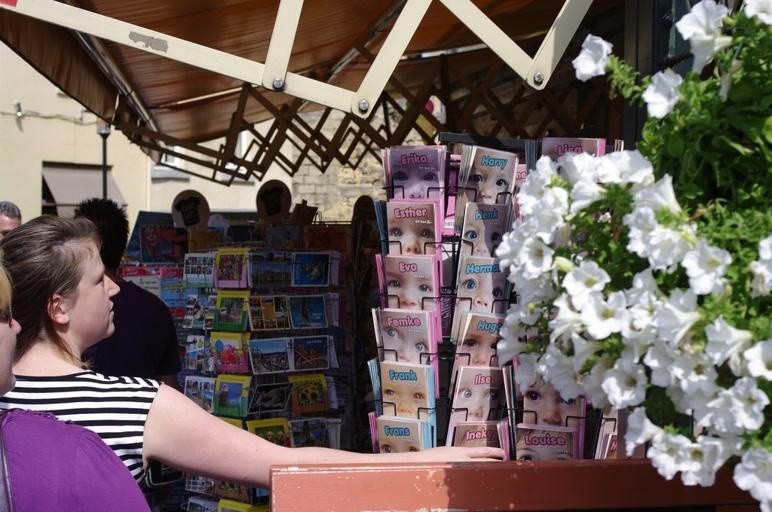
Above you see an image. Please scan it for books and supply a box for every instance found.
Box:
[120,202,343,508]
[366,138,628,460]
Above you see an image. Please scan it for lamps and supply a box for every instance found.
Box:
[14,101,23,122]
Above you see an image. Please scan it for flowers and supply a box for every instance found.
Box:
[491,0,772,512]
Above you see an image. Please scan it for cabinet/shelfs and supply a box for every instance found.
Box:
[365,133,627,459]
[120,209,359,510]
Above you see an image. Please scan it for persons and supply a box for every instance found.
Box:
[71,198,182,392]
[1,200,23,238]
[1,252,152,512]
[1,213,507,511]
